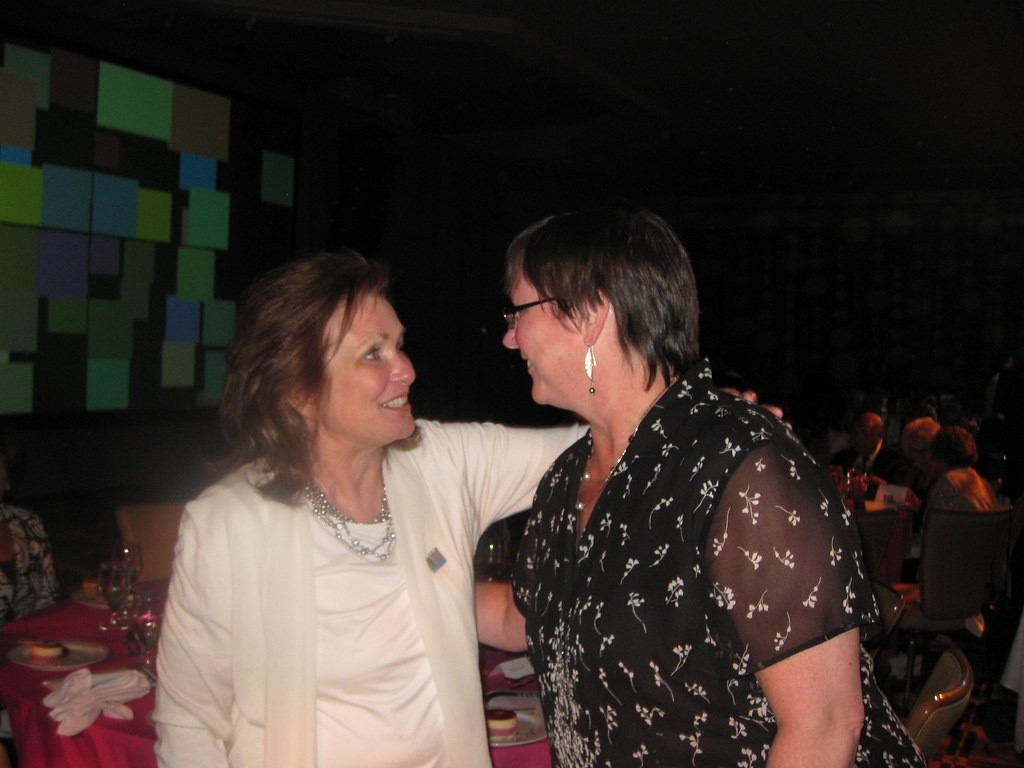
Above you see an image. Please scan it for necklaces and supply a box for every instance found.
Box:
[575,375,675,512]
[301,478,392,528]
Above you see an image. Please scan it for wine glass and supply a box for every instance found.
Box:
[133,591,162,674]
[99,544,130,631]
[117,540,145,616]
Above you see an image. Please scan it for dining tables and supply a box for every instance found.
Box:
[1,575,554,767]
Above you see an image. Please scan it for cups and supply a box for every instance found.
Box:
[847,467,867,496]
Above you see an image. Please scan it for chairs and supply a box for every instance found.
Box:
[900,635,976,758]
[896,504,1013,718]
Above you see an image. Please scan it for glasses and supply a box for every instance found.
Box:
[910,447,931,458]
[503,296,560,330]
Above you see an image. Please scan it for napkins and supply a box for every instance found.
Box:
[40,666,153,738]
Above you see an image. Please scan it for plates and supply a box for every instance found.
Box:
[482,687,547,747]
[8,641,110,670]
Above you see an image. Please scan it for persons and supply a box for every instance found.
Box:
[150,251,795,768]
[470,202,931,768]
[1,462,58,767]
[882,426,997,629]
[825,408,913,490]
[892,414,944,513]
